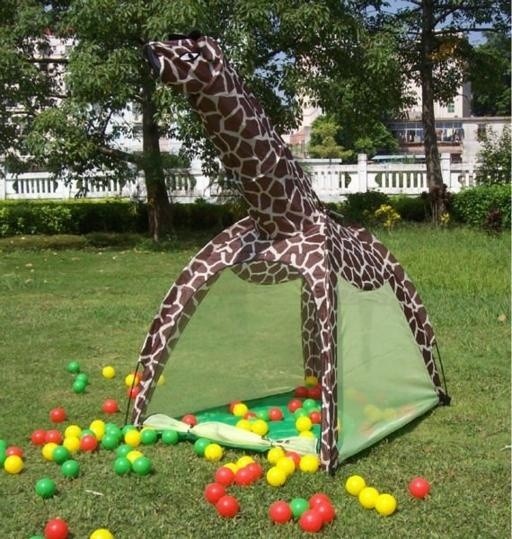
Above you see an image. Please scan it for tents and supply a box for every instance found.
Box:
[122,34,450,476]
